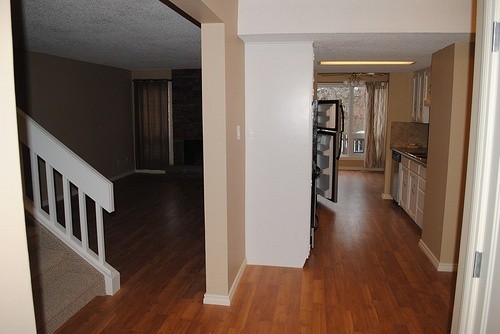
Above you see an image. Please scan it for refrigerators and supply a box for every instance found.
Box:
[312,99,345,204]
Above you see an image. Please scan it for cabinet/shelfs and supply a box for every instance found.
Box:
[400,154,417,227]
[417,164,427,229]
[410,68,430,124]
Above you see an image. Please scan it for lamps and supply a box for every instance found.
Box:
[342,72,367,86]
